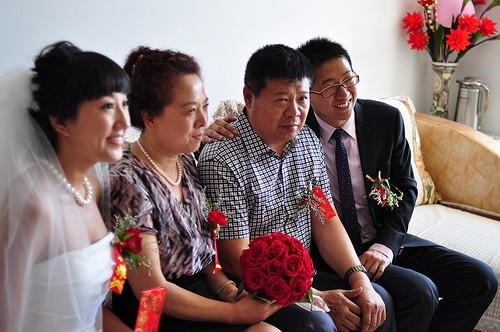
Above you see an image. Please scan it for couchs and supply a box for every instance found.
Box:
[213,97,500,332]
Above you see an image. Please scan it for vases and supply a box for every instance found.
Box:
[430,62,459,119]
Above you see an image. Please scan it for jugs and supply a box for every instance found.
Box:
[455,76,489,130]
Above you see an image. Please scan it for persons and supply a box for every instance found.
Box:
[0,40,134,332]
[193,36,498,332]
[98,46,283,332]
[198,45,396,332]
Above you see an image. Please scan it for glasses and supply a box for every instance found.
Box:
[310,70,359,98]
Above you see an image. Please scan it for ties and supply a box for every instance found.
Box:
[331,128,363,256]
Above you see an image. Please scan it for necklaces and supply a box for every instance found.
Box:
[136,138,182,186]
[37,158,93,206]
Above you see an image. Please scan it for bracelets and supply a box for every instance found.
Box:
[344,265,367,283]
[216,280,236,295]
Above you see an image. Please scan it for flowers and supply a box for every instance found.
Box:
[110,208,161,276]
[401,0,500,63]
[362,171,403,210]
[234,232,316,305]
[296,179,327,226]
[201,185,229,239]
